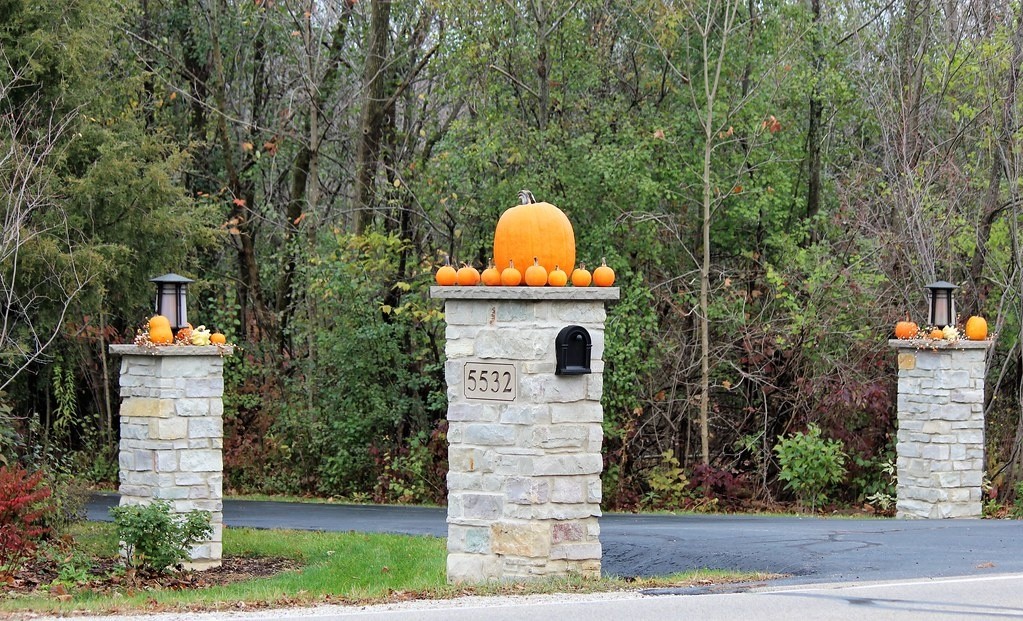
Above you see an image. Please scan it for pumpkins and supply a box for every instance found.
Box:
[966,316,987,340]
[211,334,225,346]
[594,267,614,286]
[494,203,576,287]
[525,266,547,286]
[436,267,456,285]
[572,269,591,286]
[149,316,172,344]
[501,268,521,286]
[895,322,917,339]
[481,269,500,285]
[932,330,943,339]
[548,271,567,286]
[457,267,480,285]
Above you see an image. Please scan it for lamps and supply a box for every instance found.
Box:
[148,273,194,336]
[924,281,960,334]
